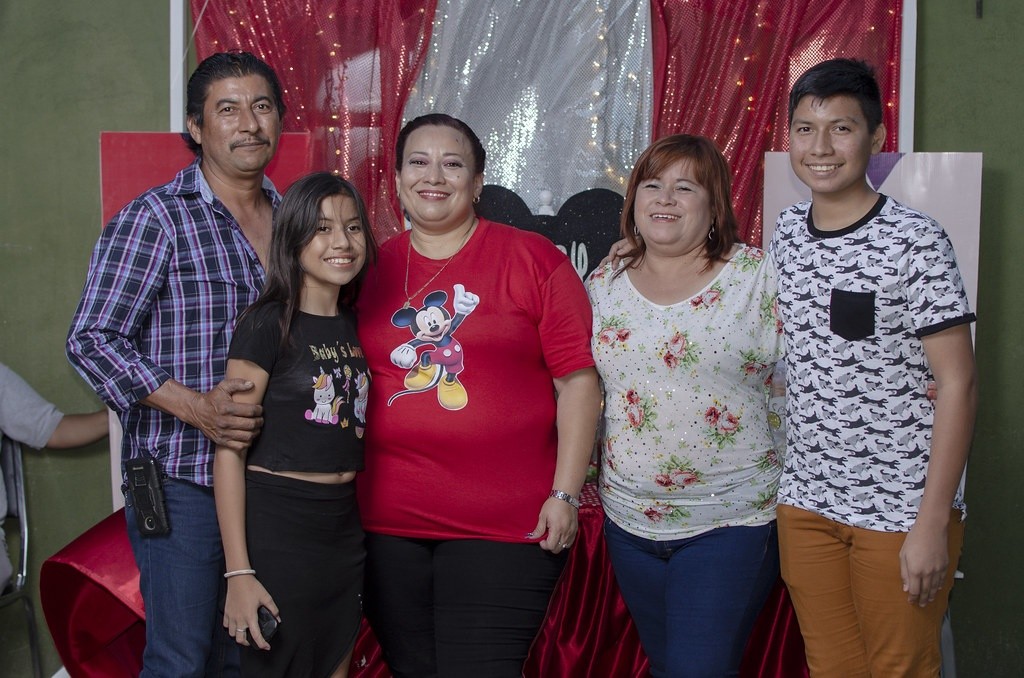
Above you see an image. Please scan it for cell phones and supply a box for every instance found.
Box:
[125,457,172,537]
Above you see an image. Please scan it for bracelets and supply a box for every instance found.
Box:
[548,489,579,511]
[223,569,256,578]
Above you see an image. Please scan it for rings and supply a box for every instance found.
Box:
[559,541,568,548]
[236,629,246,632]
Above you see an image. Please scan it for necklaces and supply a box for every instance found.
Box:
[402,230,468,310]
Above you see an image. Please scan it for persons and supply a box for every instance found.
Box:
[0,51,976,678]
